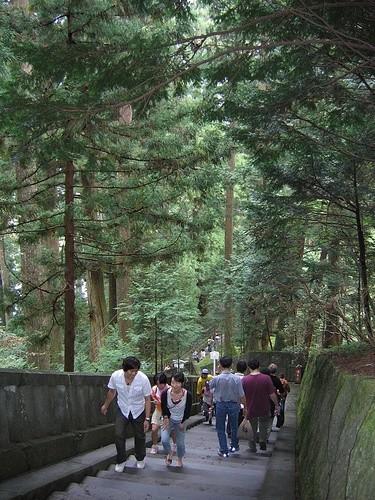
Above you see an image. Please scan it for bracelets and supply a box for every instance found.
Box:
[145,417,150,421]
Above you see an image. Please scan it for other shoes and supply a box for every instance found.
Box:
[115,459,128,473]
[209,418,212,425]
[151,444,159,455]
[248,440,256,451]
[218,448,229,457]
[205,414,209,421]
[175,462,183,467]
[228,446,240,452]
[137,458,146,469]
[259,441,266,450]
[271,426,280,431]
[171,441,177,453]
[165,451,173,466]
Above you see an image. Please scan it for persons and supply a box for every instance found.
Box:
[165,357,189,370]
[209,355,248,458]
[242,358,280,452]
[256,363,290,442]
[197,368,216,424]
[225,360,247,438]
[102,357,152,472]
[193,334,221,362]
[150,373,178,454]
[159,371,192,466]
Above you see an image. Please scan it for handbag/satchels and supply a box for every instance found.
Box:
[235,419,254,442]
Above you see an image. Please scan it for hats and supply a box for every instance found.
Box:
[202,369,209,374]
[205,380,209,386]
